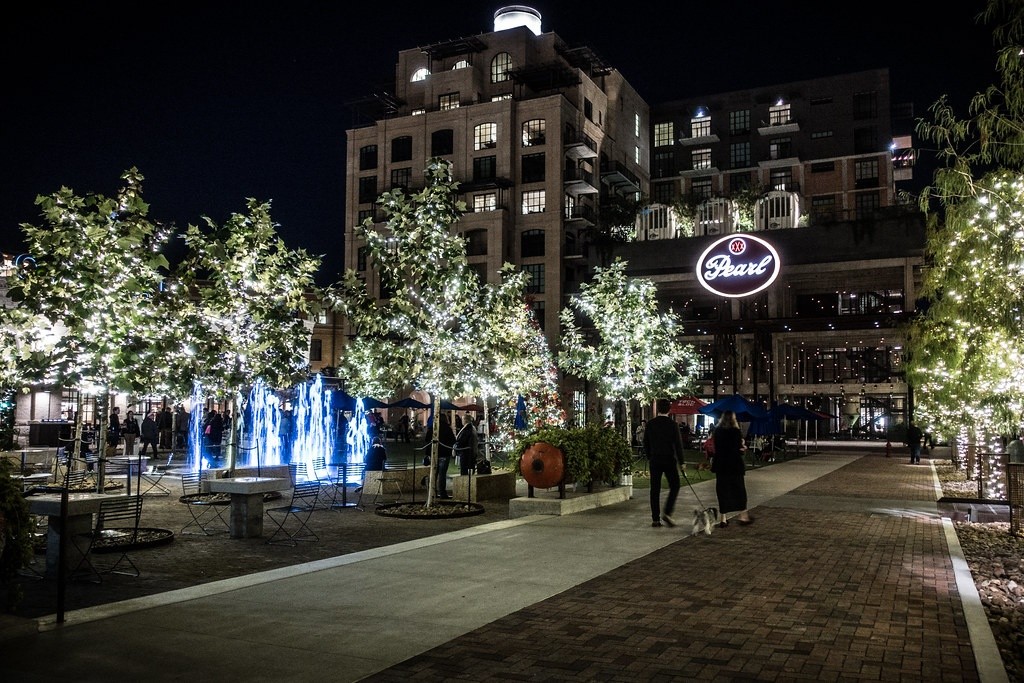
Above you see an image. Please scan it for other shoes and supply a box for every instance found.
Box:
[440,494,453,499]
[720,520,729,528]
[354,486,362,492]
[916,460,919,465]
[659,513,676,526]
[652,522,665,527]
[739,520,753,525]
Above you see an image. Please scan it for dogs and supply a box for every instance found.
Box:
[693,507,718,536]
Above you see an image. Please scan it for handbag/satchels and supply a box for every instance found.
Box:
[703,435,715,460]
[205,425,211,434]
[477,460,491,474]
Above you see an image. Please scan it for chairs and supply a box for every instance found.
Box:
[632,453,651,479]
[71,498,143,584]
[86,453,98,461]
[179,474,231,535]
[313,458,344,504]
[141,453,174,496]
[58,456,95,489]
[265,480,320,544]
[373,459,407,506]
[684,460,701,480]
[288,462,328,511]
[62,470,85,489]
[741,438,784,468]
[333,464,364,513]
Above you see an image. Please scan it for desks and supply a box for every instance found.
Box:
[31,493,136,575]
[203,476,290,539]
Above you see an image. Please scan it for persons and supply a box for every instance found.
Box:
[355,437,387,493]
[141,407,191,459]
[109,407,121,450]
[202,408,231,461]
[454,414,494,475]
[425,415,456,498]
[642,399,685,527]
[905,421,923,464]
[703,423,716,472]
[710,411,754,527]
[751,433,786,462]
[122,411,140,455]
[636,419,646,454]
[240,407,411,465]
[923,433,935,450]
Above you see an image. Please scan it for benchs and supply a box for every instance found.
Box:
[362,464,430,494]
[206,464,296,490]
[456,470,519,504]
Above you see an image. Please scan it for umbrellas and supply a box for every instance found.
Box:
[328,390,501,412]
[512,395,528,432]
[669,394,836,455]
